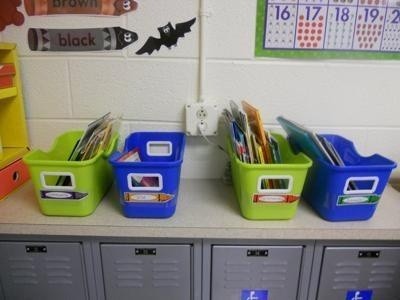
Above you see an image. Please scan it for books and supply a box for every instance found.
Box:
[57,113,121,188]
[118,149,158,187]
[221,100,285,190]
[276,115,358,191]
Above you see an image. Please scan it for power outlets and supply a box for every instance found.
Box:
[186,102,217,138]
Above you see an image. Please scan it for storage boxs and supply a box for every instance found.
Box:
[108,132,185,220]
[286,134,398,222]
[23,129,120,217]
[228,135,313,220]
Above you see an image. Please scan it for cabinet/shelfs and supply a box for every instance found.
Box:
[0,178,399,299]
